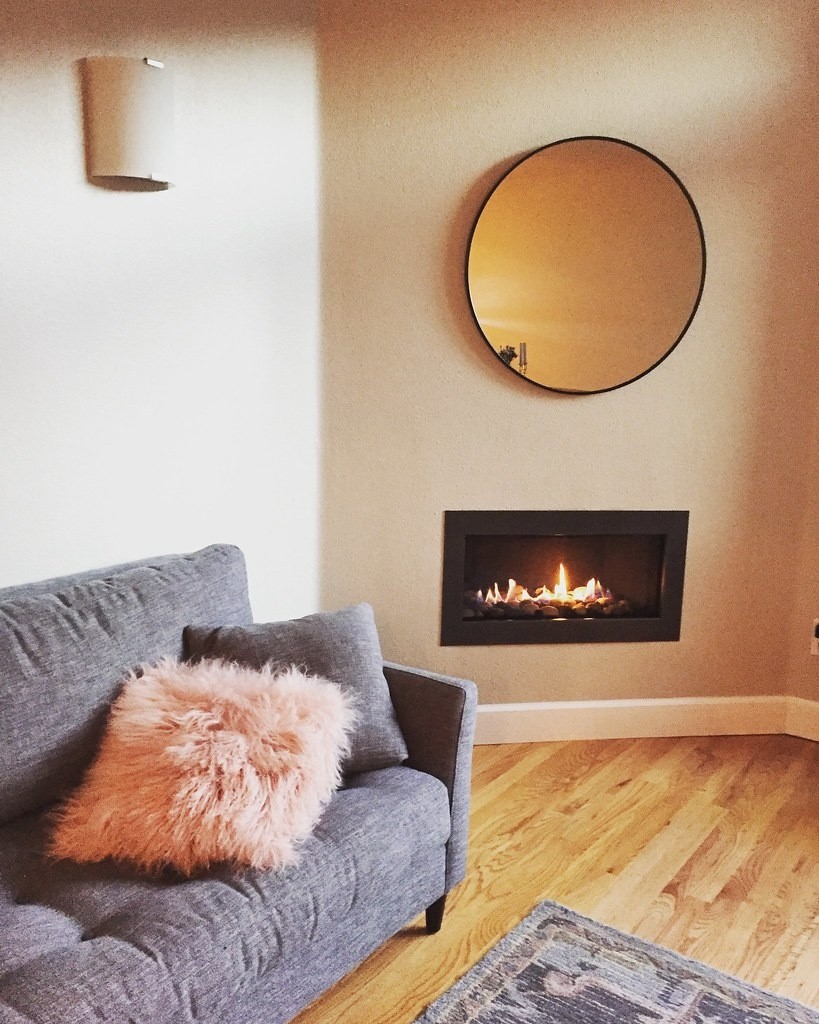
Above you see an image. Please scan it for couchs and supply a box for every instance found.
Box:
[0,555,480,1024]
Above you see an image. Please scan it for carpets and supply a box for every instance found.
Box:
[413,899,819,1024]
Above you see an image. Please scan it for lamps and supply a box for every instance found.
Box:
[83,54,177,189]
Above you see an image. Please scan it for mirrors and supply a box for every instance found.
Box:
[463,136,708,395]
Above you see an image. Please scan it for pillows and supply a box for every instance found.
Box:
[37,647,364,877]
[0,543,254,826]
[184,601,409,777]
